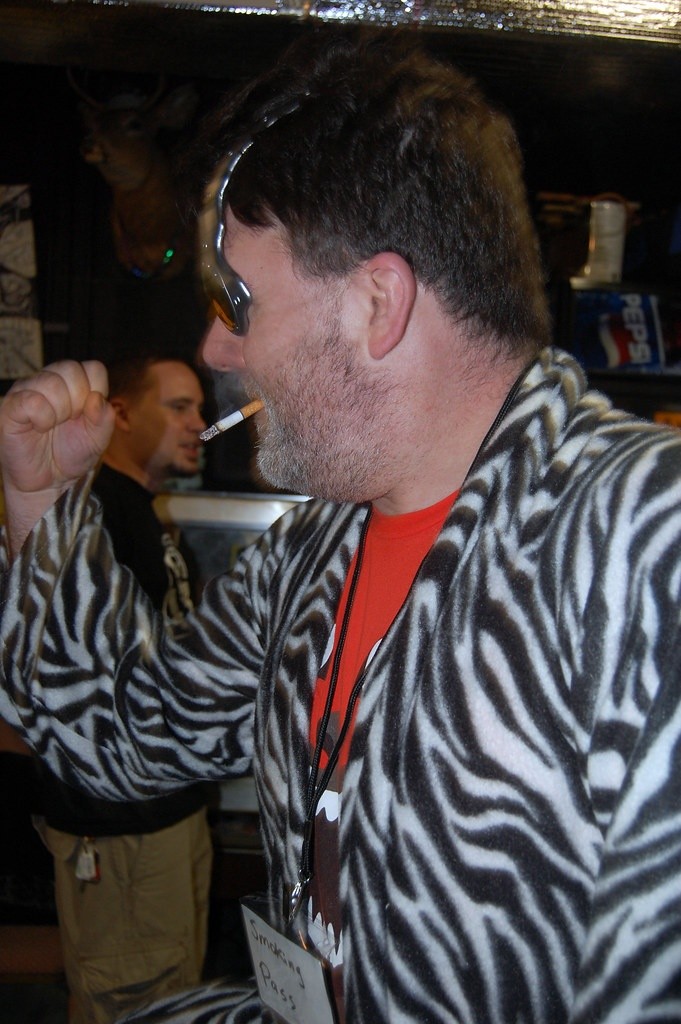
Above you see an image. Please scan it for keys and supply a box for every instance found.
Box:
[63,833,102,894]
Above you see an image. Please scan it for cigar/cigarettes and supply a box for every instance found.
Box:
[199,400,263,442]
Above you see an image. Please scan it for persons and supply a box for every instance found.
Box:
[0,51,681,1024]
